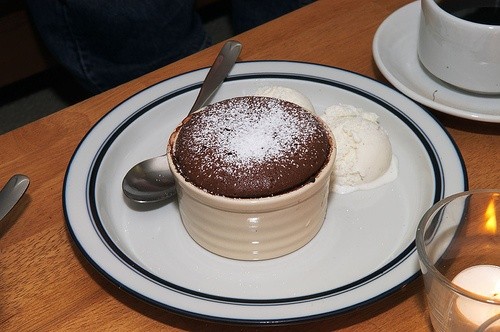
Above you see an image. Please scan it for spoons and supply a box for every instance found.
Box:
[122,38,243,204]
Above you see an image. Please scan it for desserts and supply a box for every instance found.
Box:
[172,95,330,198]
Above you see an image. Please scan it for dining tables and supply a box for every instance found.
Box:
[0,0,500,332]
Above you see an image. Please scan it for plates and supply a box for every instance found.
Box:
[372,0,499,123]
[63,59,469,330]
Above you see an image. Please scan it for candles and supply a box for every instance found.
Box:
[447,264,500,332]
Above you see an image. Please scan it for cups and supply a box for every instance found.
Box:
[415,189,500,332]
[416,0,499,95]
[166,96,337,262]
